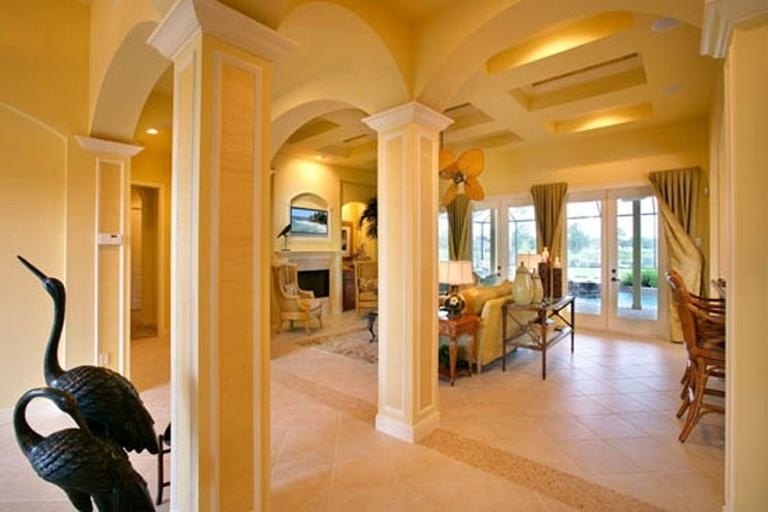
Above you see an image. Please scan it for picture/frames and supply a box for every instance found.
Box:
[341,221,353,259]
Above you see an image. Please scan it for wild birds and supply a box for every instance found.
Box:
[15,254,160,456]
[11,385,157,512]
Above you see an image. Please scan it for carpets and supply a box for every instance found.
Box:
[294,325,469,383]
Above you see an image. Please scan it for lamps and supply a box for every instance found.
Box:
[439,260,473,320]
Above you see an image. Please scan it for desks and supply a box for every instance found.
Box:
[502,297,576,380]
[436,312,479,387]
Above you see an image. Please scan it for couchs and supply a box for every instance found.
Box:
[439,285,539,372]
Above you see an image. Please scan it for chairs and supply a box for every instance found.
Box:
[270,264,323,337]
[664,270,727,445]
[353,261,378,317]
